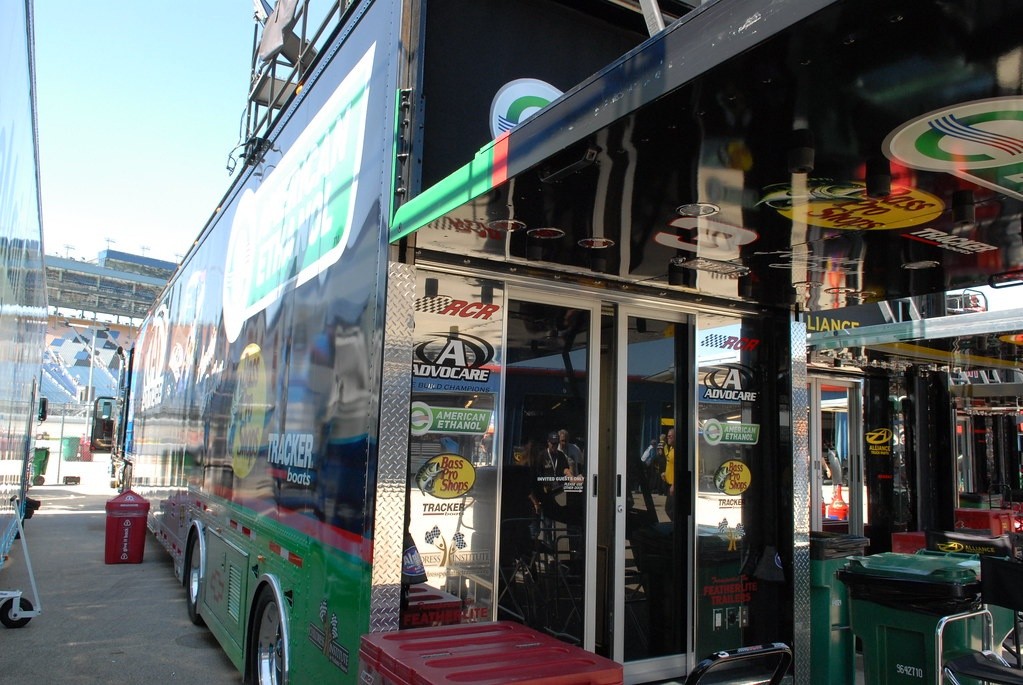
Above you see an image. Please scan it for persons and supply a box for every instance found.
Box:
[821,457,830,491]
[521,429,583,550]
[639,428,676,522]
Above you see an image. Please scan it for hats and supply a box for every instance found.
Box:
[548,432,560,444]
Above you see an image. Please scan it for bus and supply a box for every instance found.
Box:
[1,1,52,574]
[112,1,1023,685]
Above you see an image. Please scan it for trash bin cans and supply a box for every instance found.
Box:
[809,531,871,685]
[833,550,983,685]
[32,447,52,486]
[104,490,150,564]
